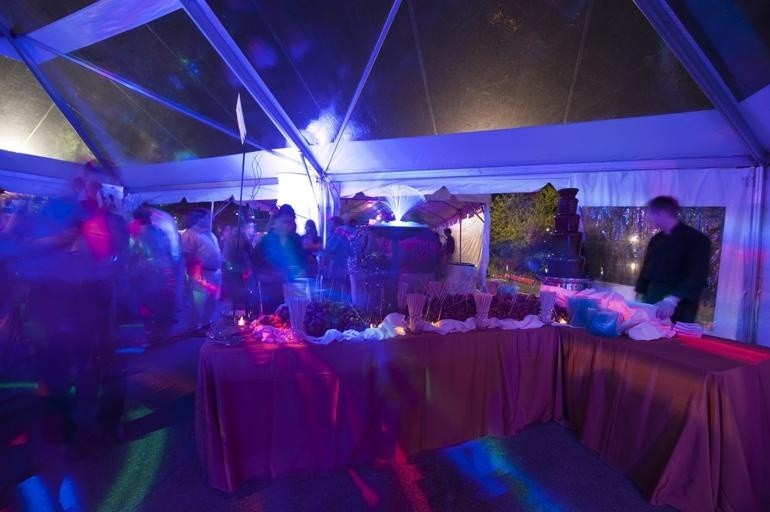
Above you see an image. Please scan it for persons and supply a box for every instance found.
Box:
[634,194,713,324]
[0,168,458,342]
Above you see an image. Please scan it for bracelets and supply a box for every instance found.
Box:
[663,297,679,308]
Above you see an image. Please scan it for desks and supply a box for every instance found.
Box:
[205,314,560,492]
[558,305,758,510]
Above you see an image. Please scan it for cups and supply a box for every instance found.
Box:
[474,292,493,329]
[539,291,557,326]
[285,295,310,341]
[406,294,425,331]
